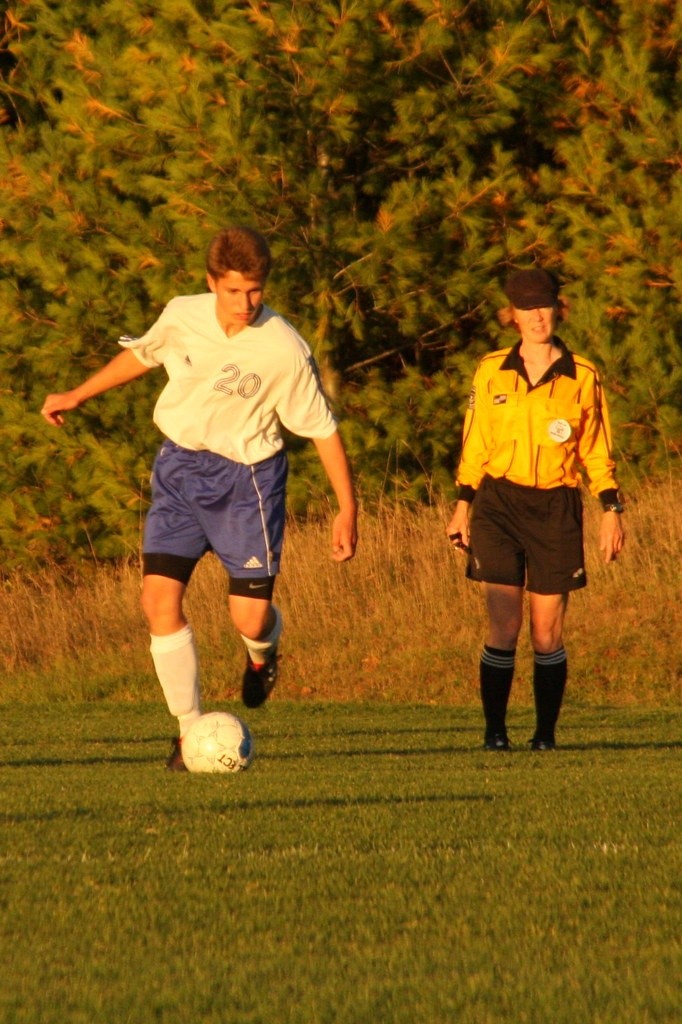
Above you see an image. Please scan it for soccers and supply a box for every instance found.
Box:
[180,710,256,774]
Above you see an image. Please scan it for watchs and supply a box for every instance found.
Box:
[605,503,624,513]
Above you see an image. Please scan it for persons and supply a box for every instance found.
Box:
[40,225,360,772]
[449,269,629,751]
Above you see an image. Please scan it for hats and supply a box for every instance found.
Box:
[504,268,559,308]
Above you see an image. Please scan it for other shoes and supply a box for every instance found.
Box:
[531,731,554,751]
[165,737,187,773]
[241,650,279,709]
[484,728,509,750]
